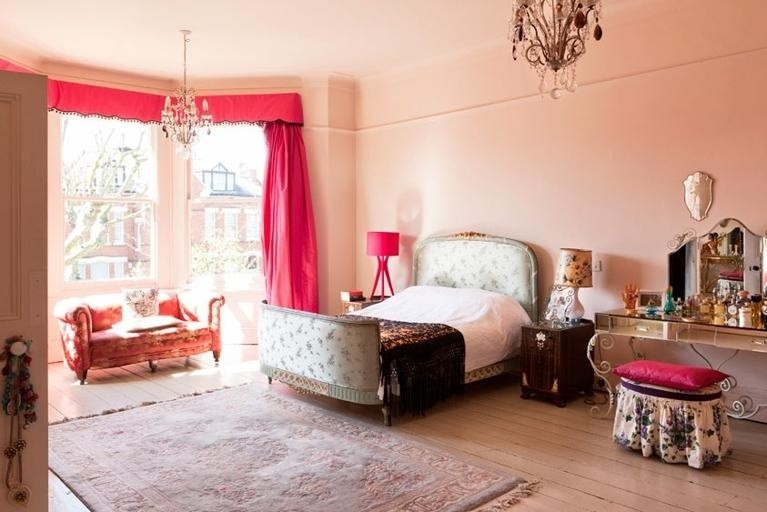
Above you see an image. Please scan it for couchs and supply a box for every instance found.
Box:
[53,288,226,385]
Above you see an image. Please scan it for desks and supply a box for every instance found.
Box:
[584,307,764,420]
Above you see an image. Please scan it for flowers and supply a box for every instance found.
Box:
[1,334,38,423]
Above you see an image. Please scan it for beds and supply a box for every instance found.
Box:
[258,230,541,428]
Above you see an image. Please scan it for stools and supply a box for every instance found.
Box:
[613,377,727,469]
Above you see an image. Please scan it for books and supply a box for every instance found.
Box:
[341,290,366,302]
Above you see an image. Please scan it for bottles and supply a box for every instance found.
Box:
[664,285,674,314]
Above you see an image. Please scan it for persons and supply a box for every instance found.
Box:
[703,232,721,256]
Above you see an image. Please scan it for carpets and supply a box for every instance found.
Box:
[46,379,537,512]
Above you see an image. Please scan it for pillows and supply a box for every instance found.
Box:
[110,286,184,334]
[612,359,731,390]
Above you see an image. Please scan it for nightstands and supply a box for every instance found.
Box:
[520,319,597,407]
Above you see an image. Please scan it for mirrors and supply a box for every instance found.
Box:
[664,216,767,305]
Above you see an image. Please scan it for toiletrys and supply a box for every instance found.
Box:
[662,286,763,330]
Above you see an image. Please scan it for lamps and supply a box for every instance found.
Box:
[554,246,594,319]
[158,29,214,155]
[366,230,401,302]
[507,0,605,103]
